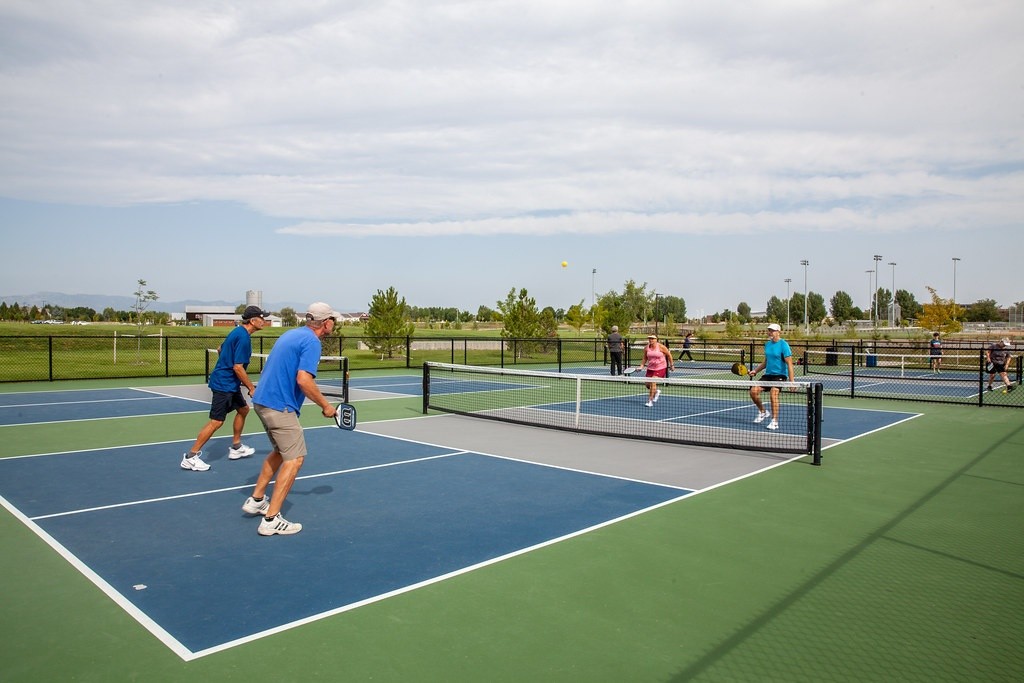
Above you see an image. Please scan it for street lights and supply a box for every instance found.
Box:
[866,270,875,329]
[799,259,810,327]
[952,257,961,322]
[784,278,792,332]
[592,269,597,306]
[655,293,663,336]
[873,255,883,328]
[888,263,897,329]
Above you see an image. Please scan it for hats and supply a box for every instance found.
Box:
[1001,339,1010,346]
[648,335,657,339]
[305,302,341,320]
[242,306,270,319]
[767,323,781,332]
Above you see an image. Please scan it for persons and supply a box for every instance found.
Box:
[636,333,674,407]
[748,323,796,430]
[678,333,696,362]
[180,305,265,471]
[928,333,944,375]
[985,337,1017,393]
[607,325,625,375]
[242,302,338,536]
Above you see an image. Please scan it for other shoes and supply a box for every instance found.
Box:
[986,385,994,392]
[690,360,695,362]
[1007,386,1017,393]
[678,359,682,362]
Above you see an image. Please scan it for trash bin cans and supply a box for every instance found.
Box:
[825,347,836,366]
[866,353,877,366]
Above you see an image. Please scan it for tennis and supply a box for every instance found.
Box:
[1002,390,1007,395]
[561,261,568,268]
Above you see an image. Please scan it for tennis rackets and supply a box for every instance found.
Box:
[322,402,357,431]
[731,363,756,377]
[623,367,641,374]
[986,362,995,372]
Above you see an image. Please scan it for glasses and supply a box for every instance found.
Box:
[322,317,335,322]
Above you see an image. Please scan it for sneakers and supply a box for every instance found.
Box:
[766,419,779,430]
[644,401,653,406]
[258,512,302,535]
[653,390,661,403]
[228,444,255,459]
[180,451,210,471]
[242,495,270,515]
[753,409,770,423]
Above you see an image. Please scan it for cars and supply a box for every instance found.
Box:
[30,319,64,324]
